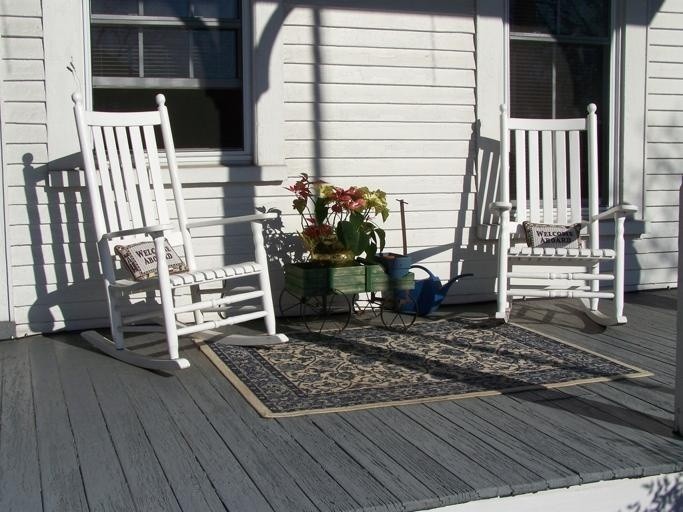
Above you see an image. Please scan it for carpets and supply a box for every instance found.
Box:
[189,314,656,419]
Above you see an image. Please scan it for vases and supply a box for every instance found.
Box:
[308,253,355,267]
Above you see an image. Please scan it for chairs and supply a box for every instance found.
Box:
[492,101,640,329]
[67,90,290,372]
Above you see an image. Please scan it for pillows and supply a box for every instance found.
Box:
[519,220,581,252]
[112,237,189,283]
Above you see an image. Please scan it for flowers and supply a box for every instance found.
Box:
[284,172,391,253]
